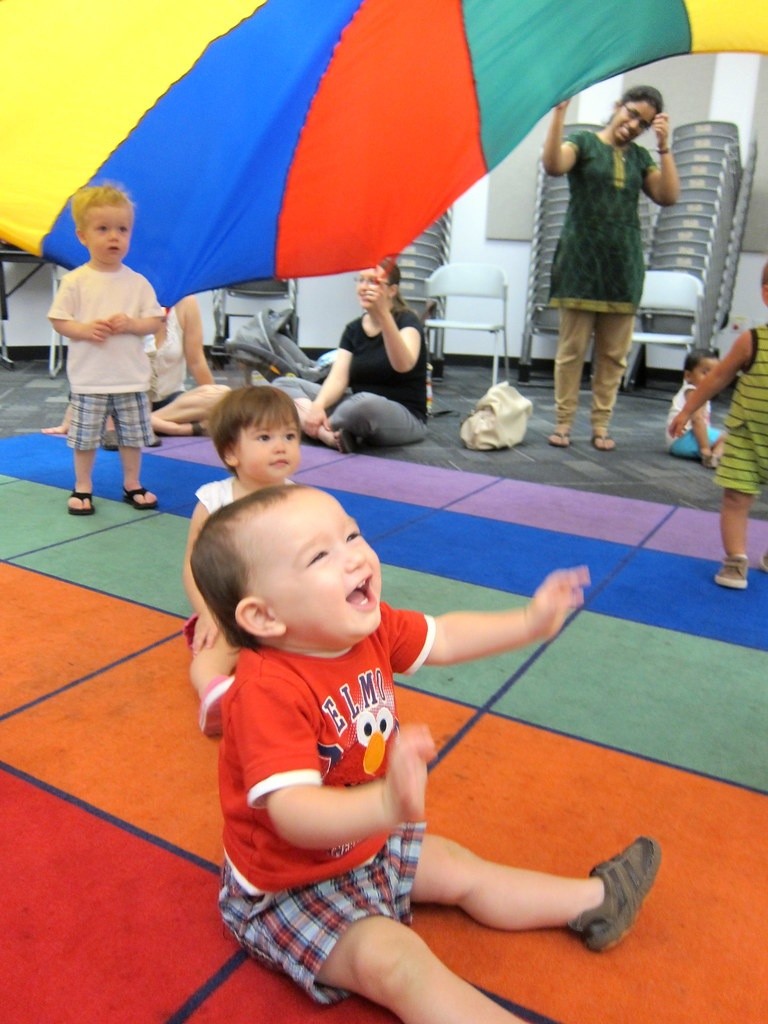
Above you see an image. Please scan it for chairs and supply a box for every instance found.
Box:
[394,203,510,388]
[208,278,299,372]
[517,121,758,400]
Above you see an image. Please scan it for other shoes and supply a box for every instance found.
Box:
[198,676,239,737]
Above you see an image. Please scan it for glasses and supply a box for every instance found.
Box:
[353,275,373,285]
[624,103,650,130]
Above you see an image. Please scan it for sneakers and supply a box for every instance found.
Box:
[570,836,661,952]
[716,554,750,588]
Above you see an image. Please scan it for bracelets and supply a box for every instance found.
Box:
[656,145,671,154]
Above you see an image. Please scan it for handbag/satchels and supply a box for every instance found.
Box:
[460,381,533,451]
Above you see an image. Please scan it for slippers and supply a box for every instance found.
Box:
[592,433,614,450]
[548,430,570,447]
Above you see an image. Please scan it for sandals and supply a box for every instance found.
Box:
[122,484,157,507]
[334,428,355,454]
[68,488,94,515]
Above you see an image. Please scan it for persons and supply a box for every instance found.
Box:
[191,486,665,1023]
[46,185,167,516]
[182,385,307,735]
[268,256,431,455]
[668,261,768,588]
[41,293,232,450]
[540,81,681,450]
[664,348,726,468]
